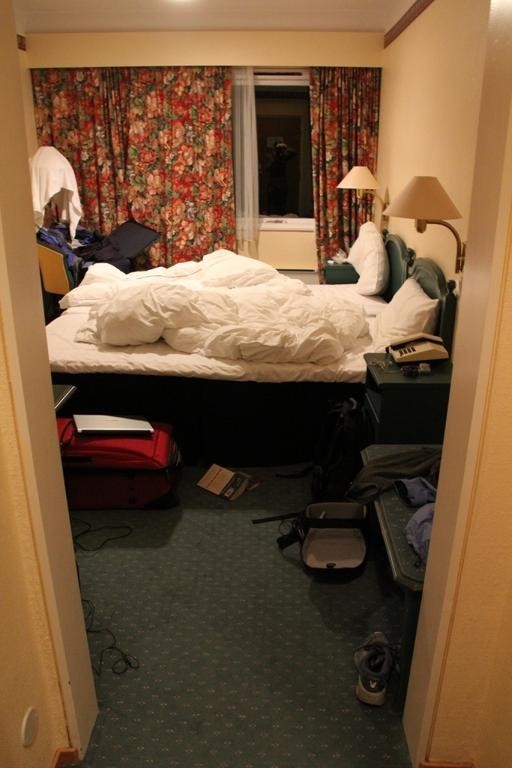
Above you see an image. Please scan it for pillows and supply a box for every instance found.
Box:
[347,219,438,344]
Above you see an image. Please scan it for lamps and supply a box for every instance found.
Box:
[337,162,468,270]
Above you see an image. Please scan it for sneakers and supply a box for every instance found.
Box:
[355,632,393,706]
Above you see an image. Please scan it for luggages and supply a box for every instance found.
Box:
[55,416,182,510]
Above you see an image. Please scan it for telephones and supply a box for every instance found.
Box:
[388,332,449,364]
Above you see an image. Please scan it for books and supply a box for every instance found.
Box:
[196,464,261,501]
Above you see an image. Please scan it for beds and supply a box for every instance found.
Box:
[45,236,451,462]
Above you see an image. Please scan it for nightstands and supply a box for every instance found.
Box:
[362,352,447,453]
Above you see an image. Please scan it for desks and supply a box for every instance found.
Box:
[361,437,441,700]
